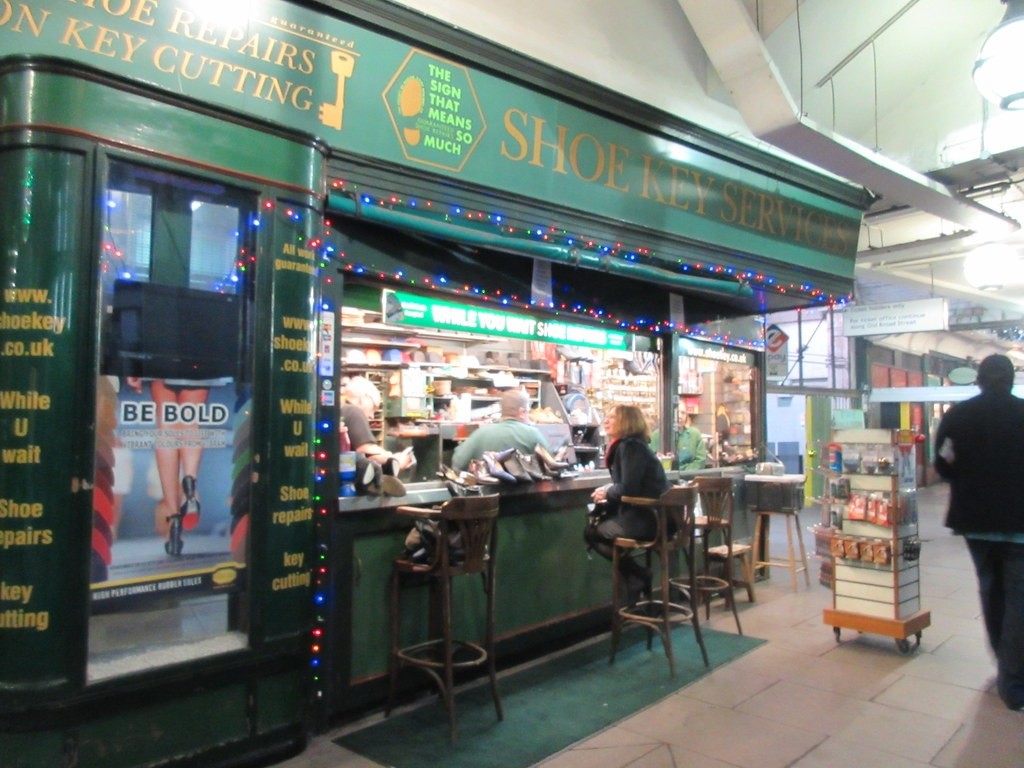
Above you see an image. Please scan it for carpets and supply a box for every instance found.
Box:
[329,619,769,768]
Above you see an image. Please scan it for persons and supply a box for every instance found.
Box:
[932,354,1024,710]
[338,376,417,476]
[650,401,707,472]
[583,405,677,606]
[127,377,232,556]
[452,390,554,476]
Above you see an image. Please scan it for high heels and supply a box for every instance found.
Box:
[164,512,183,557]
[435,443,570,487]
[179,475,200,532]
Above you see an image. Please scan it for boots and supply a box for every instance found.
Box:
[619,558,653,601]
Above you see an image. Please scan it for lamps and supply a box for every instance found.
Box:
[972,0,1024,111]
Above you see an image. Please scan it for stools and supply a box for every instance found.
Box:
[607,483,712,672]
[382,492,501,751]
[669,477,743,643]
[704,541,755,609]
[749,510,810,592]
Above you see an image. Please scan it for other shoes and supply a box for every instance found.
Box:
[357,456,410,498]
